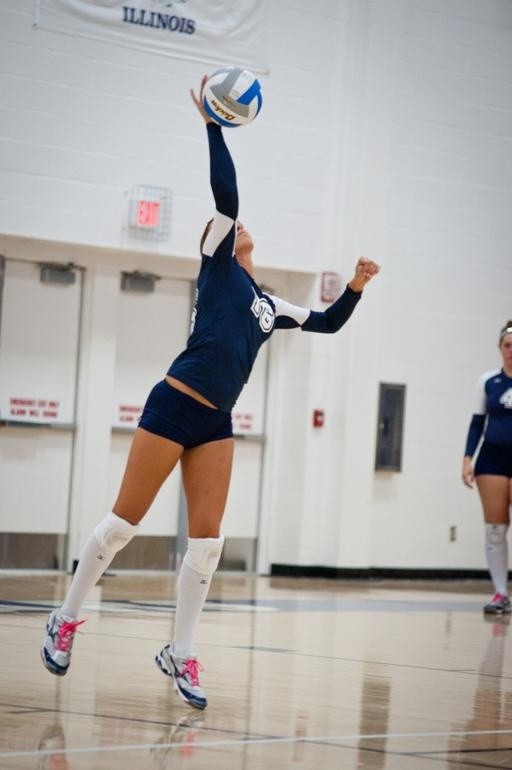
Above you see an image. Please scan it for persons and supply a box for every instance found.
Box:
[463,321,512,613]
[42,75,379,712]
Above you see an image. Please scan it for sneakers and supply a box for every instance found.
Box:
[41,609,78,675]
[154,643,208,710]
[484,593,511,614]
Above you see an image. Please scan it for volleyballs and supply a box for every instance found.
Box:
[201,66,262,126]
